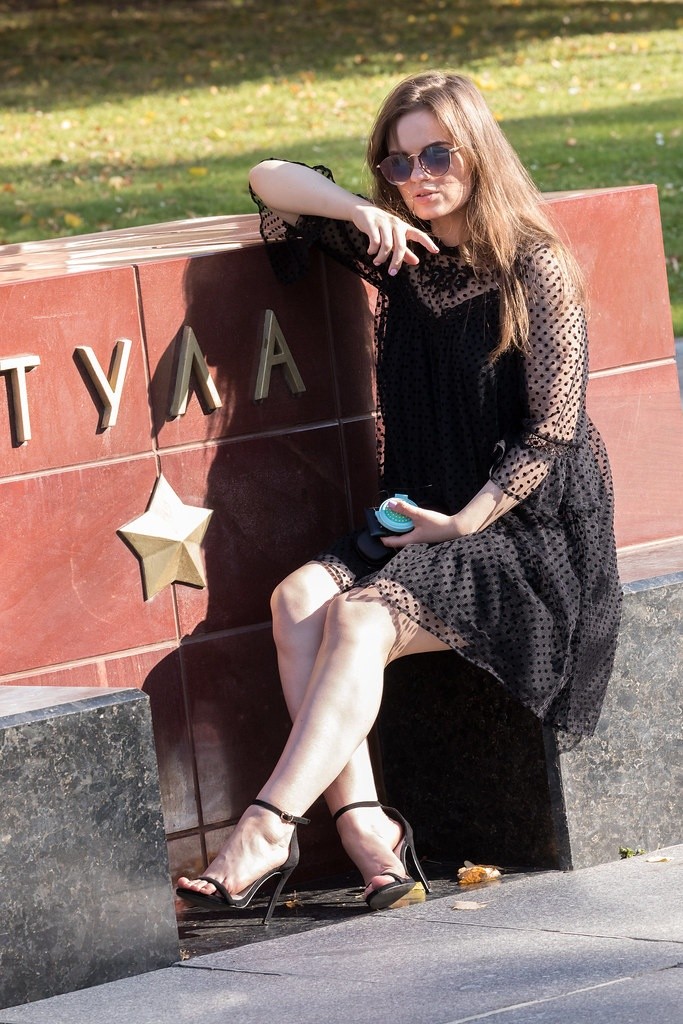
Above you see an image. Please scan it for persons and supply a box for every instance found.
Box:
[173,70,621,926]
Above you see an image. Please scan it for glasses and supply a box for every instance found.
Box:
[377,144,470,185]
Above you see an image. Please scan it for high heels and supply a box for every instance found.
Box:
[333,801,433,910]
[176,798,311,926]
